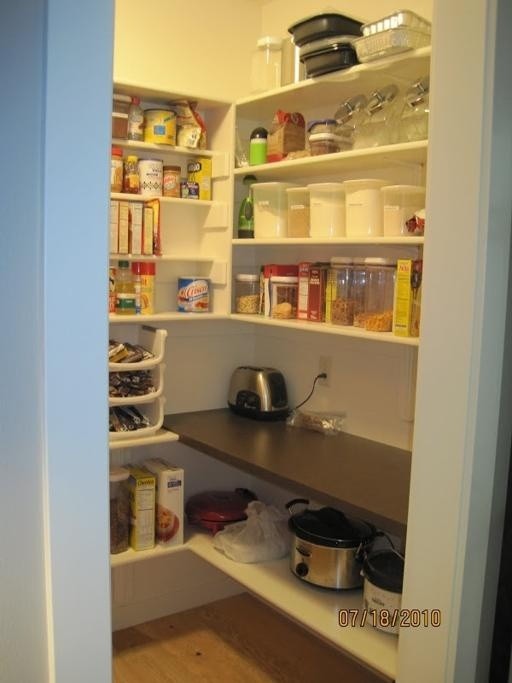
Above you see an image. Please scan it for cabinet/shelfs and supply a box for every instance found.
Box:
[109,323,167,436]
[109,427,392,681]
[110,46,434,345]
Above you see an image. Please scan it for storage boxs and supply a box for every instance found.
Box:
[393,260,422,337]
[123,457,184,551]
[186,156,212,200]
[249,179,426,238]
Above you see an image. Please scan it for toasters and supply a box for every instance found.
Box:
[226,364,290,423]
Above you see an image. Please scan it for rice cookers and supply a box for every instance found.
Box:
[284,499,376,591]
[359,550,401,633]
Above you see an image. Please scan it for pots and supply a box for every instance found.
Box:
[185,487,258,537]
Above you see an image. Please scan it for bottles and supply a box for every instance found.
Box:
[248,127,268,166]
[365,257,395,332]
[111,149,124,192]
[351,259,369,329]
[253,36,306,95]
[334,77,429,151]
[234,272,262,316]
[116,261,137,315]
[329,256,353,326]
[128,97,143,141]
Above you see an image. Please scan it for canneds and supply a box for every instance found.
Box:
[143,108,178,147]
[270,276,299,319]
[138,158,212,200]
[235,273,260,314]
[324,256,397,333]
[177,277,210,313]
[111,146,124,194]
